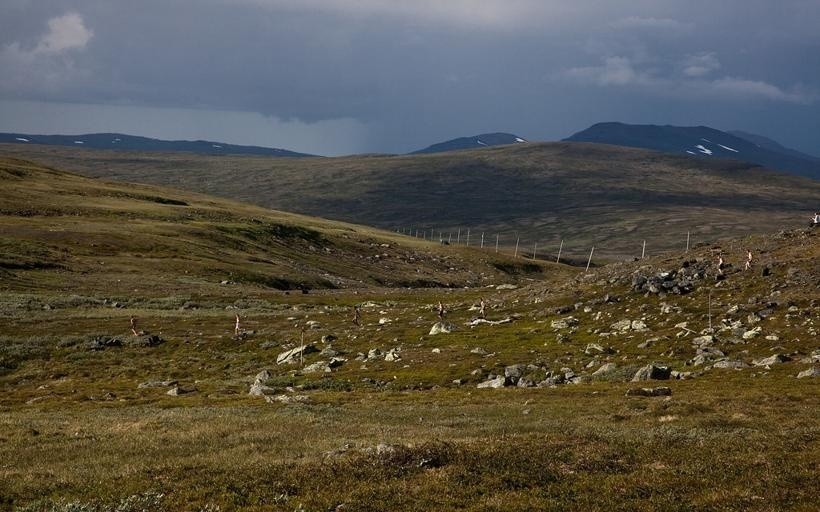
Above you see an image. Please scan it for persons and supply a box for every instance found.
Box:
[438,300,444,322]
[235,313,240,335]
[811,212,820,226]
[717,255,723,274]
[479,297,488,319]
[744,249,753,271]
[352,306,361,327]
[131,315,139,336]
